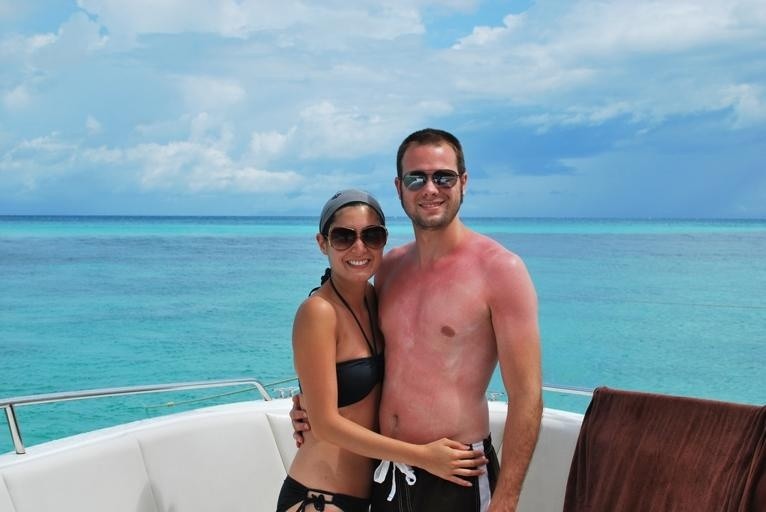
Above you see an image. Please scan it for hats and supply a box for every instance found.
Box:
[319,189,386,234]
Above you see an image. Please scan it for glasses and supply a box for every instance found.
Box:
[323,224,388,251]
[400,169,461,191]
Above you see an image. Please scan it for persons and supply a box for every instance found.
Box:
[270,187,490,511]
[289,127,543,511]
